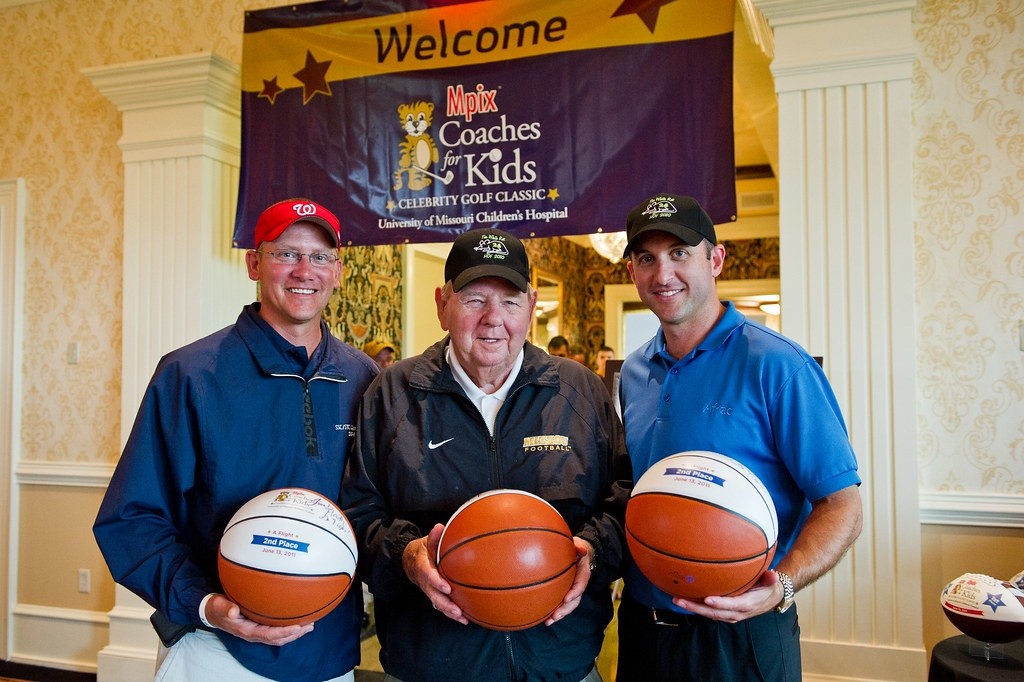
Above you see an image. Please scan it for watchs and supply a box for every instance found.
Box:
[771,569,794,614]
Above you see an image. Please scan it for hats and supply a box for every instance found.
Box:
[364,338,395,359]
[622,192,717,263]
[447,229,531,294]
[253,198,341,255]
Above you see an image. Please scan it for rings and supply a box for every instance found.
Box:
[433,604,437,609]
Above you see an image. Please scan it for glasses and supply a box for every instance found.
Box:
[256,249,339,266]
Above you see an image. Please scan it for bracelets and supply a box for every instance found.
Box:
[199,593,218,628]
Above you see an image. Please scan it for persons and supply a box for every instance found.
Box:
[596,347,614,377]
[364,339,394,368]
[569,346,585,364]
[548,336,571,358]
[615,193,863,681]
[333,229,633,682]
[92,198,382,682]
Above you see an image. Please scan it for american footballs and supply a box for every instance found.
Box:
[940,572,1023,644]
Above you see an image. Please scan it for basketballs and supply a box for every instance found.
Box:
[435,488,576,632]
[216,487,359,626]
[624,450,780,602]
